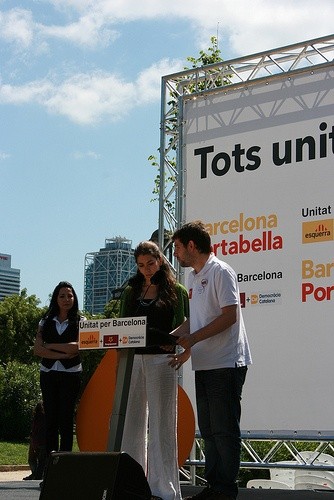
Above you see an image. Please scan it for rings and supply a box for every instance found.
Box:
[175,358,178,360]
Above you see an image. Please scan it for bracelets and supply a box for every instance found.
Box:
[178,355,185,362]
[42,341,47,348]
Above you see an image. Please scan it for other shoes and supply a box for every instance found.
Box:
[183,485,238,500]
[23,473,41,480]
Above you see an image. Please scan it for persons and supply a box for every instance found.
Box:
[174,226,253,500]
[23,282,87,479]
[107,242,191,500]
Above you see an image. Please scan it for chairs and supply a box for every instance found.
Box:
[247,451,334,490]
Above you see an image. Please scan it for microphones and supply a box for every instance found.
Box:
[134,271,164,317]
[109,278,138,318]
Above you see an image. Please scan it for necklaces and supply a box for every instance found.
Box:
[139,293,156,305]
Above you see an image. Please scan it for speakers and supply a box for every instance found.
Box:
[38,451,152,500]
